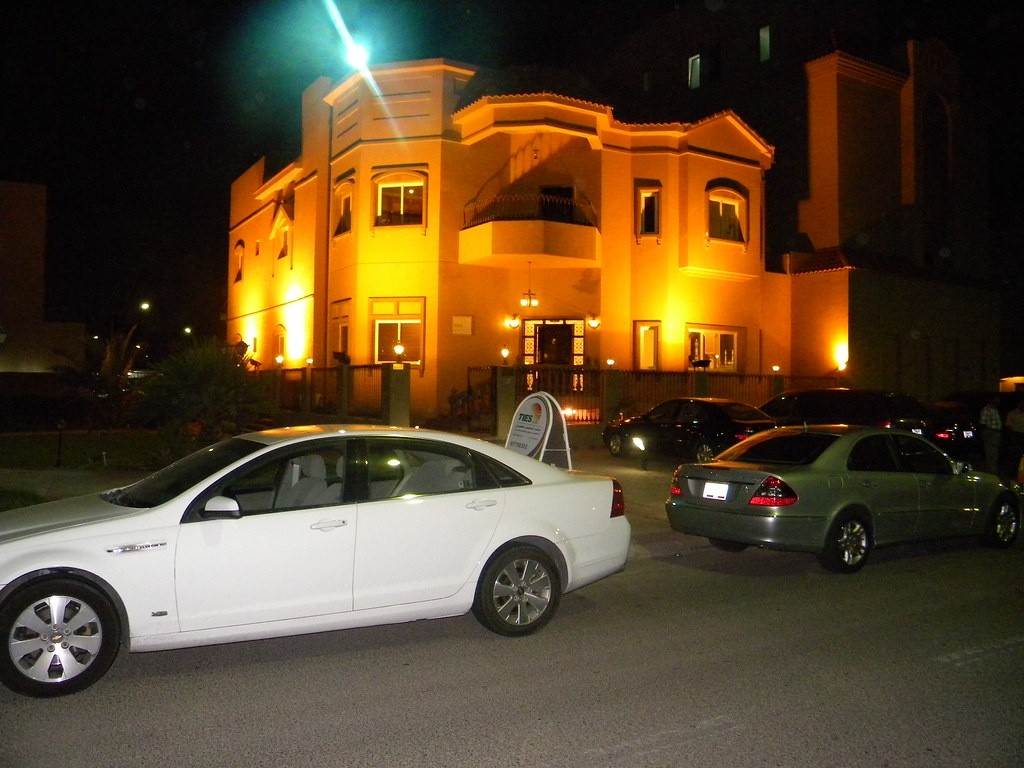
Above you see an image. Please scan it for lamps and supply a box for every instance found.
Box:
[275,355,285,367]
[306,357,314,366]
[502,345,510,366]
[520,261,538,308]
[607,357,615,368]
[394,340,404,363]
[588,312,600,329]
[509,312,520,329]
[772,364,780,374]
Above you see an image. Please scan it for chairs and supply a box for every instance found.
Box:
[285,454,328,506]
[317,455,344,502]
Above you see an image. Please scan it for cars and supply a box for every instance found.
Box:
[664,425,1024,575]
[601,398,782,463]
[759,387,986,473]
[0,425,633,700]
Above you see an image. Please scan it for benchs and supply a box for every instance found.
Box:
[392,459,464,495]
